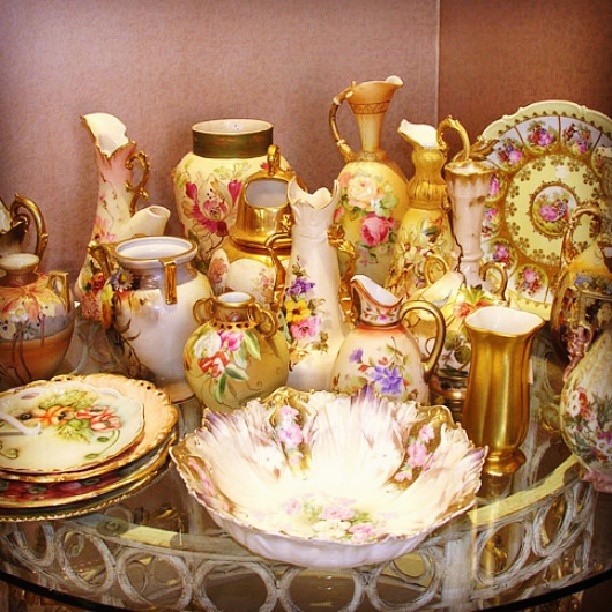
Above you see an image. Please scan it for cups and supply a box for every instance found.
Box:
[459,307,545,476]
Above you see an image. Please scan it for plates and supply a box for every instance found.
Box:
[3,373,177,520]
[472,97,612,315]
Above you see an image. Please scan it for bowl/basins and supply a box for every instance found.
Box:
[168,386,489,569]
